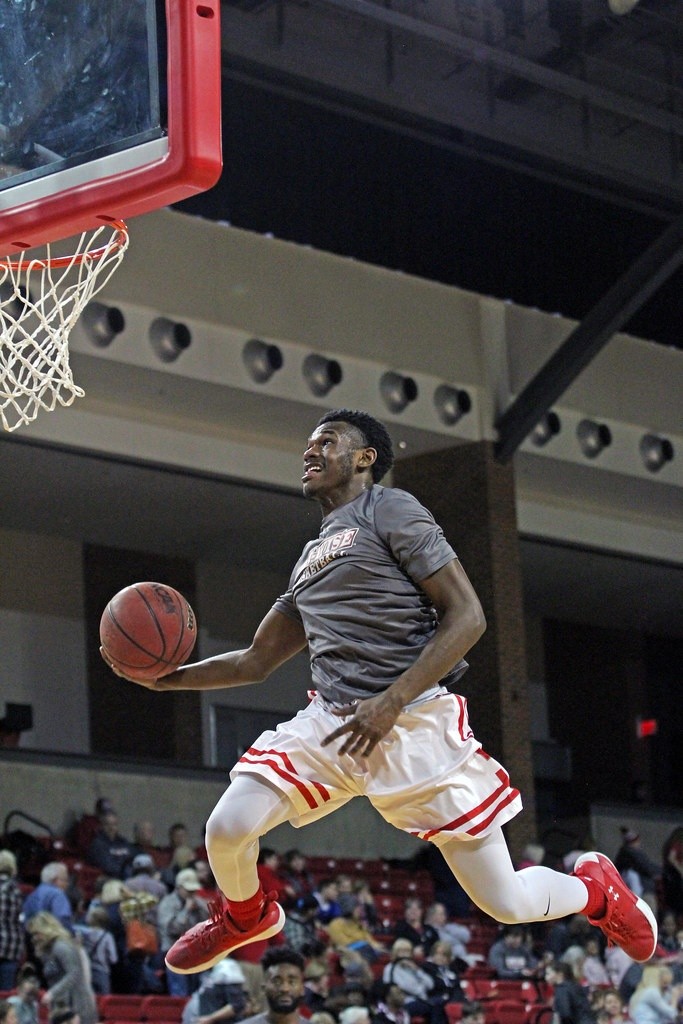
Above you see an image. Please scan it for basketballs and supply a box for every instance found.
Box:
[100,581,199,681]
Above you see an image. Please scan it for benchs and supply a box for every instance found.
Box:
[1,836,682,1024]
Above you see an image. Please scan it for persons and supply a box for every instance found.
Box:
[94,410,661,976]
[0,797,683,1024]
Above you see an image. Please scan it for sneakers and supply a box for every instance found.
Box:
[164,890,286,975]
[570,851,658,964]
